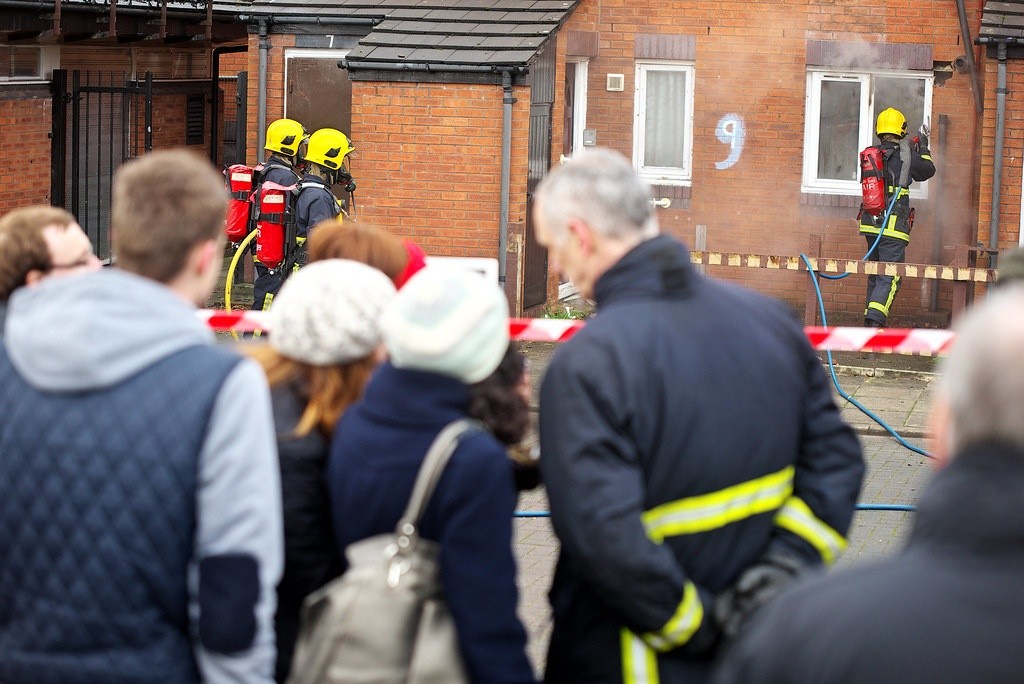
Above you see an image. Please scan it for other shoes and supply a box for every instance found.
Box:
[864,318,882,327]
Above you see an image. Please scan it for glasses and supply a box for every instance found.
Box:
[39,245,92,271]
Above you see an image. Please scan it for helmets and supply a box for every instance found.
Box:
[264,119,310,156]
[876,106,907,139]
[304,128,356,170]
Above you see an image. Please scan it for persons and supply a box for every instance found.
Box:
[0,205,104,305]
[330,262,539,684]
[856,107,936,327]
[288,128,359,272]
[248,217,427,684]
[717,249,1024,684]
[250,119,310,275]
[0,147,284,684]
[532,144,864,684]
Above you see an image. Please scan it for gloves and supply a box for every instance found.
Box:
[710,557,801,646]
[917,131,928,147]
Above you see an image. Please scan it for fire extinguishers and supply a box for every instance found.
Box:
[860,147,885,216]
[224,165,253,249]
[255,180,287,275]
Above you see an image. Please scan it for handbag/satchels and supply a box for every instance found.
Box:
[288,417,485,684]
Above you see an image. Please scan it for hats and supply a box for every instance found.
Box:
[378,264,510,386]
[266,258,397,366]
